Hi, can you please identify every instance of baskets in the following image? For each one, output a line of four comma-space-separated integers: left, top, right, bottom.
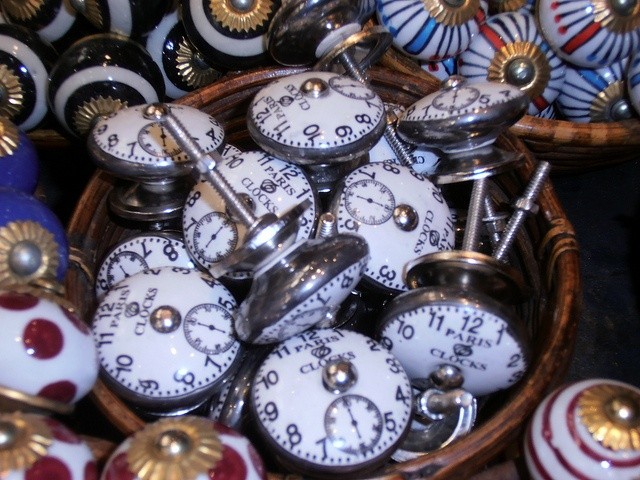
60, 68, 580, 480
363, 1, 639, 176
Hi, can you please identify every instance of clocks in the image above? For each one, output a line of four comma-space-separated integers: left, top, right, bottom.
211, 201, 369, 344
331, 161, 456, 294
395, 75, 530, 183
373, 287, 534, 398
93, 267, 241, 415
182, 152, 321, 281
95, 234, 194, 303
86, 103, 227, 222
252, 326, 416, 480
245, 71, 388, 165
